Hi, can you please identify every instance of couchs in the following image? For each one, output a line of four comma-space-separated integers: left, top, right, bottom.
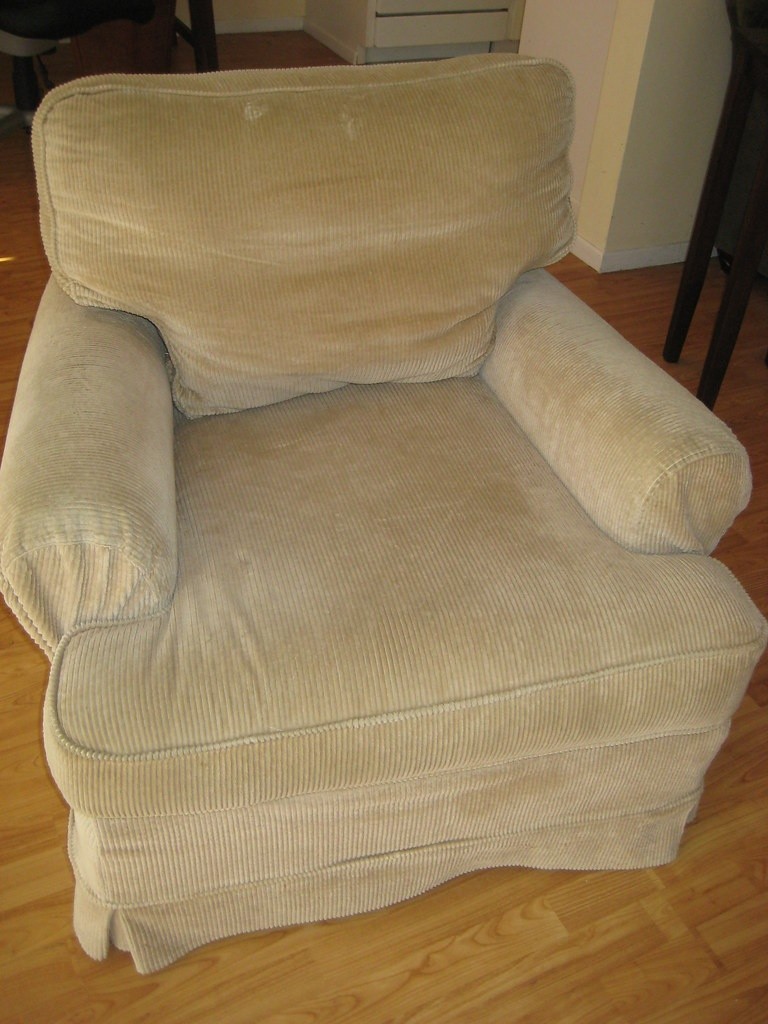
0, 52, 768, 975
